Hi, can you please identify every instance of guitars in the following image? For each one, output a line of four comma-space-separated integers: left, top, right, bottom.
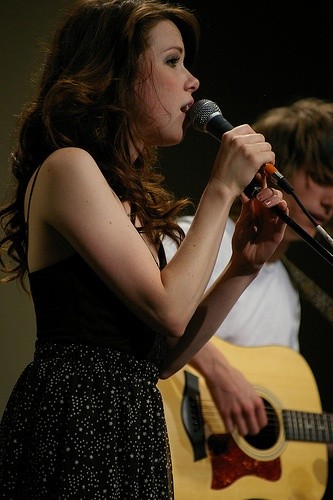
156, 337, 332, 500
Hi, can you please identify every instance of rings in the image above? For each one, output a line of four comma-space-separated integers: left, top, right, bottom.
270, 187, 278, 198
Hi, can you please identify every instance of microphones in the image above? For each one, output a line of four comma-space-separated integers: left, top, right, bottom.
187, 99, 294, 194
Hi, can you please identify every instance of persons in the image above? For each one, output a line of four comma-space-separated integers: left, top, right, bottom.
150, 100, 333, 436
0, 0, 289, 500
